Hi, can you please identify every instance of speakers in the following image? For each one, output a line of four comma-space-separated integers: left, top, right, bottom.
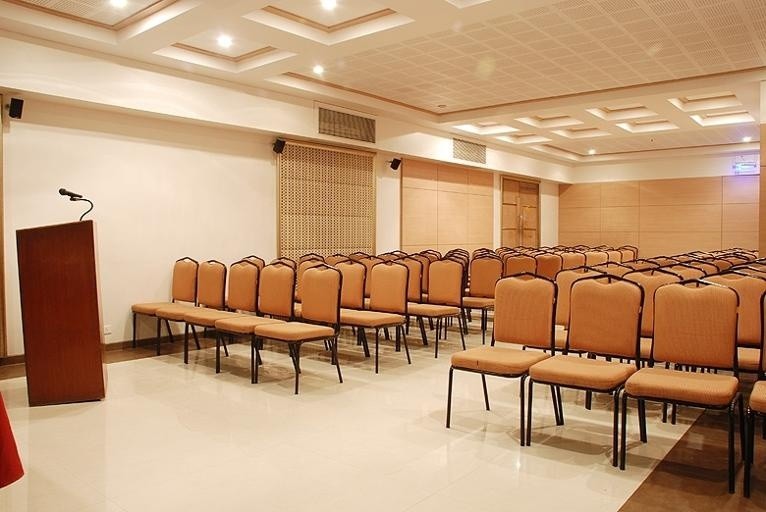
273, 137, 286, 153
8, 98, 24, 120
390, 158, 401, 170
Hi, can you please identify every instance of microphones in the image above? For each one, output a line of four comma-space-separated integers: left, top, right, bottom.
58, 187, 81, 199
